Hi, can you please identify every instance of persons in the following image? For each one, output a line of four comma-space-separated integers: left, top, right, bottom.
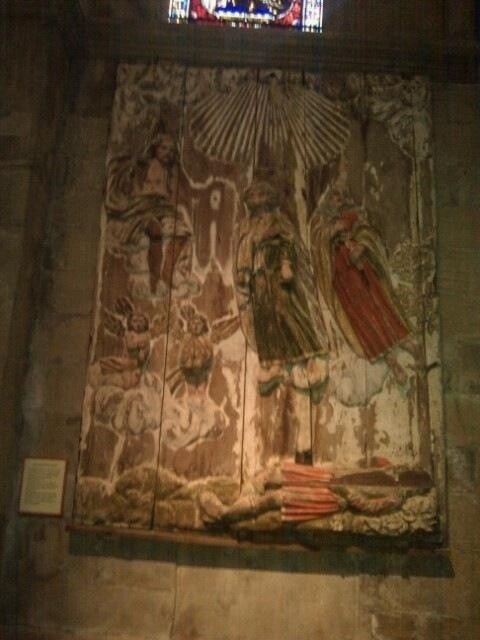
307, 182, 421, 384
166, 304, 241, 450
107, 130, 193, 294
93, 297, 174, 434
233, 182, 332, 406
198, 467, 434, 533
